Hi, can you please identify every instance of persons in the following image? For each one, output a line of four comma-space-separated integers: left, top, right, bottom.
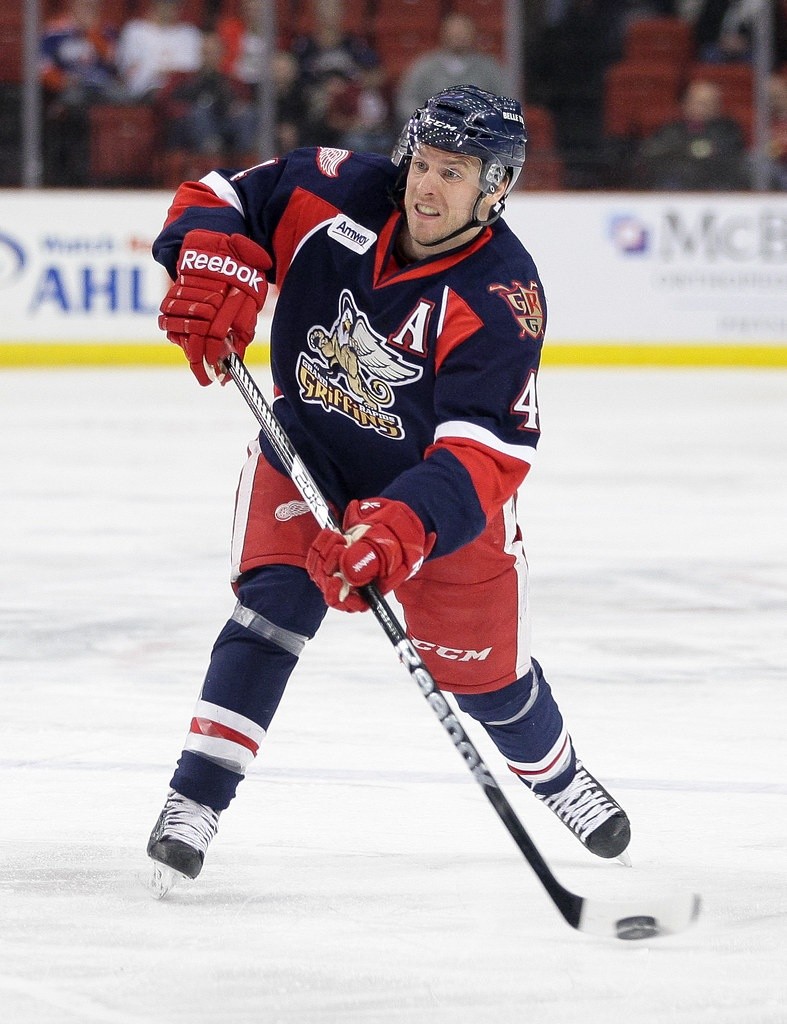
38, 0, 787, 192
148, 86, 630, 900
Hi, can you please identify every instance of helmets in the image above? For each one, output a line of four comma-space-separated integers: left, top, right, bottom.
412, 83, 526, 201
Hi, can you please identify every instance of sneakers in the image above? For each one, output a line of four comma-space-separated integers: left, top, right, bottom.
146, 790, 221, 900
524, 758, 632, 869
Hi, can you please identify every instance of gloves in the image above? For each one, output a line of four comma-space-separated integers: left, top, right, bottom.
158, 228, 273, 387
305, 495, 436, 612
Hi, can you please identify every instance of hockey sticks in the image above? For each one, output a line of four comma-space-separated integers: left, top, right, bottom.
219, 351, 707, 943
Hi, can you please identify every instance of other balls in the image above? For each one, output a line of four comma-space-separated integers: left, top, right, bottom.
616, 915, 657, 940
